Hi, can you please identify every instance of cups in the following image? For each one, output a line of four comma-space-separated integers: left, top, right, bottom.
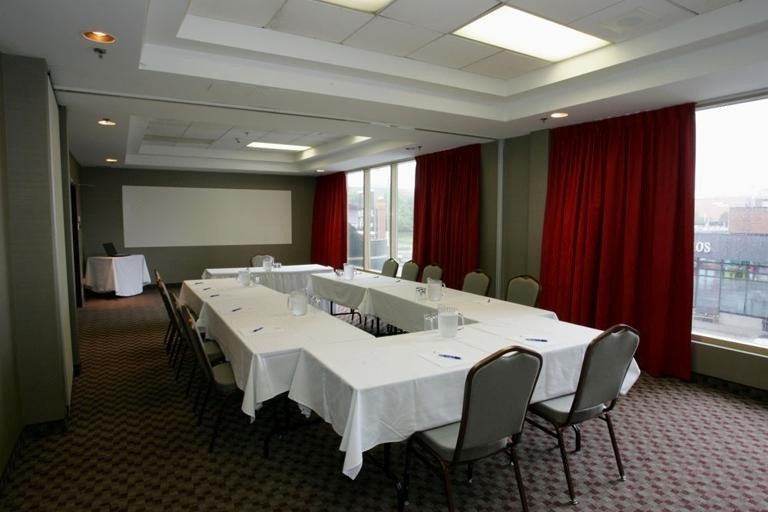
343, 263, 358, 280
263, 257, 273, 271
236, 268, 250, 286
286, 287, 309, 316
423, 313, 438, 333
335, 268, 343, 279
274, 263, 281, 270
436, 304, 464, 338
427, 277, 447, 301
416, 286, 426, 299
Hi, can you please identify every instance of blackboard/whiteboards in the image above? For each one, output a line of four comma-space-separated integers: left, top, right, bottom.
121, 185, 292, 246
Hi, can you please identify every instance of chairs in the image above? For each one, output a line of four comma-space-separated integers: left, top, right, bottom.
181, 305, 251, 455
421, 264, 443, 285
159, 282, 204, 381
504, 275, 539, 306
250, 251, 276, 268
169, 292, 224, 411
508, 324, 640, 505
154, 269, 173, 350
371, 262, 419, 332
463, 271, 490, 295
399, 345, 542, 512
351, 258, 401, 326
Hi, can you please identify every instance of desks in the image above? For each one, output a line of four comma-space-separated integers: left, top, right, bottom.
176, 276, 376, 432
307, 268, 559, 332
201, 261, 333, 312
287, 315, 640, 478
85, 254, 151, 298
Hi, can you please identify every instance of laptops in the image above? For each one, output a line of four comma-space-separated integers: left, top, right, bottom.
97, 240, 129, 256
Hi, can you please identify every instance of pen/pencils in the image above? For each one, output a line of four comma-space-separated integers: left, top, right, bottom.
488, 298, 491, 304
439, 354, 461, 359
196, 282, 203, 284
253, 327, 262, 332
211, 295, 219, 297
527, 339, 547, 342
204, 288, 211, 290
233, 308, 240, 311
